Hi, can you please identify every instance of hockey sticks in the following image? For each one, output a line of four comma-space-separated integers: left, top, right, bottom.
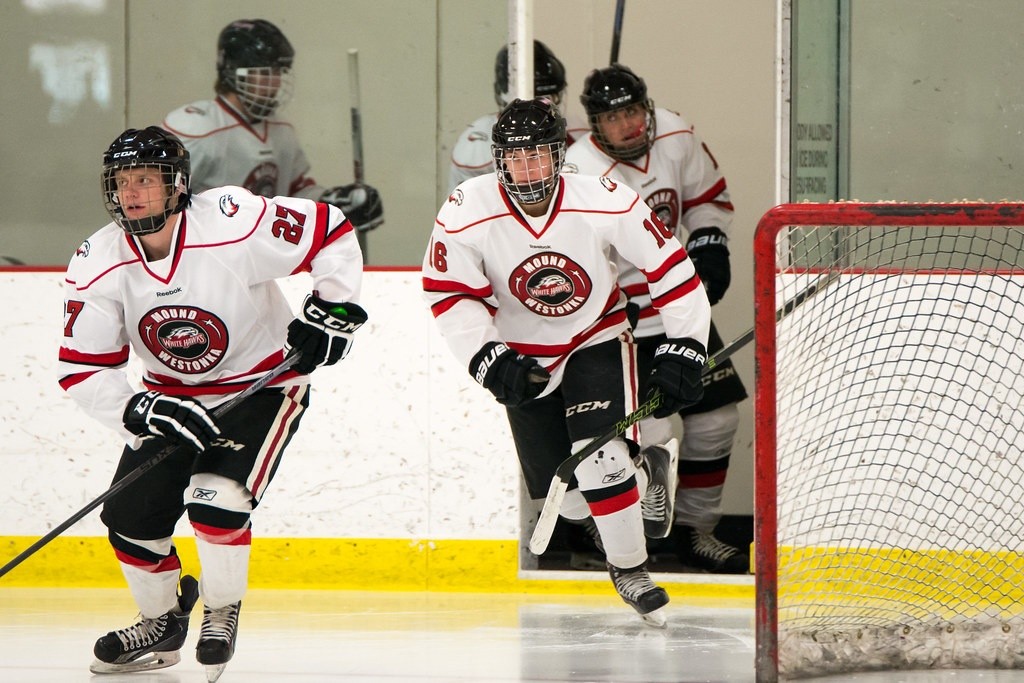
528, 269, 843, 555
346, 48, 368, 265
0, 350, 302, 577
610, 0, 625, 67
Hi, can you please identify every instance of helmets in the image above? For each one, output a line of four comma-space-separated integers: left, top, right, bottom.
490, 97, 568, 206
580, 64, 657, 159
99, 125, 194, 236
215, 19, 295, 123
494, 38, 568, 115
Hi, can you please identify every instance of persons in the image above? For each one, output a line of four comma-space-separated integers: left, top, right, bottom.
422, 97, 713, 629
450, 35, 609, 571
161, 21, 386, 230
56, 126, 368, 683
559, 63, 749, 571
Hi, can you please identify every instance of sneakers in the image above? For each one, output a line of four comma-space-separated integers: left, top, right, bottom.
632, 436, 679, 539
666, 522, 750, 575
194, 598, 241, 683
536, 511, 658, 572
594, 532, 669, 628
89, 574, 200, 673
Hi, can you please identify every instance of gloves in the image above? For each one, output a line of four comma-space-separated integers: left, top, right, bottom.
468, 342, 550, 407
644, 337, 708, 420
686, 226, 733, 306
283, 294, 369, 373
318, 185, 386, 231
122, 391, 221, 454
